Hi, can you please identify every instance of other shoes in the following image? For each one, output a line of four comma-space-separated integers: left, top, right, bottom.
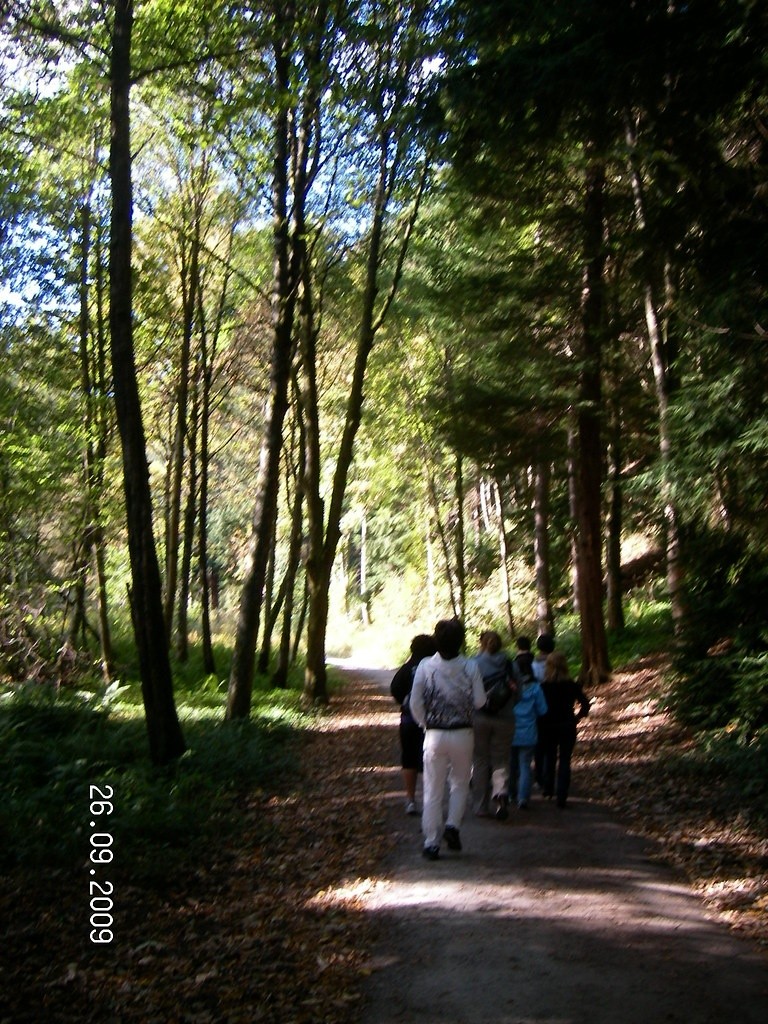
509, 796, 528, 810
541, 788, 567, 809
443, 825, 461, 850
403, 799, 417, 815
493, 802, 508, 822
421, 847, 439, 862
474, 810, 489, 818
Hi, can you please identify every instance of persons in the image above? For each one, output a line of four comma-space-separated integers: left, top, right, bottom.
506, 659, 548, 808
470, 630, 524, 819
531, 635, 554, 683
389, 633, 435, 814
411, 620, 487, 860
511, 637, 535, 659
533, 635, 589, 806
